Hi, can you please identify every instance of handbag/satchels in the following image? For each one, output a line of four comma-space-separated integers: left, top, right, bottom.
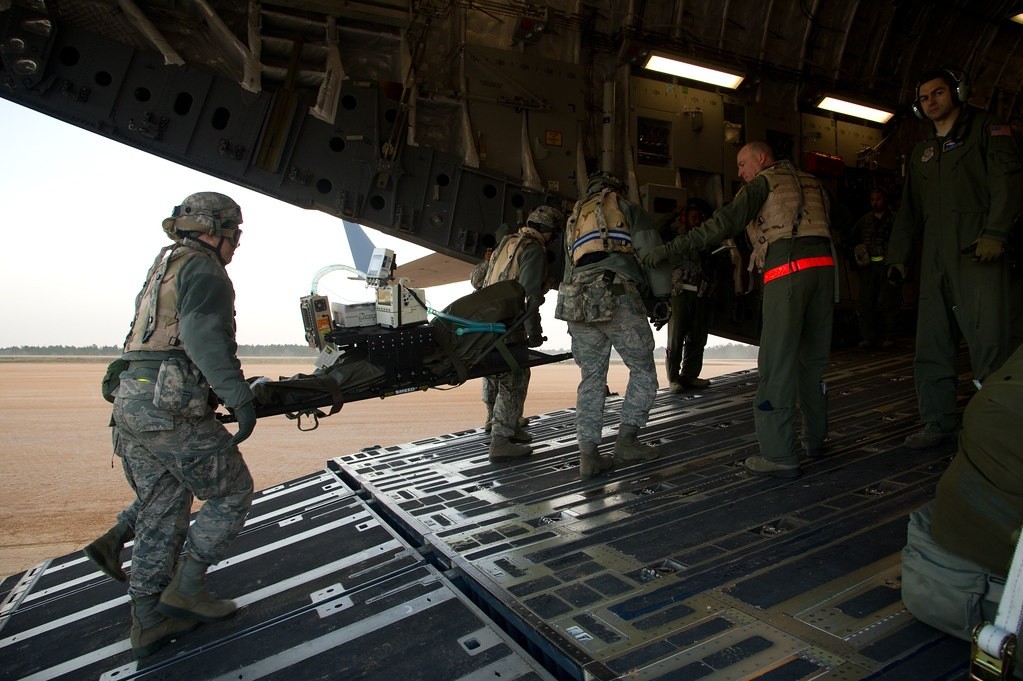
900, 507, 993, 643
555, 282, 616, 323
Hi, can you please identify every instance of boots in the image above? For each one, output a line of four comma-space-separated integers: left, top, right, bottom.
578, 442, 611, 479
156, 555, 238, 622
486, 415, 534, 441
83, 521, 133, 582
612, 425, 663, 465
487, 432, 532, 462
129, 597, 198, 659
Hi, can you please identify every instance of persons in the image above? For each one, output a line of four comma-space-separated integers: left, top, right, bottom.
641, 141, 835, 482
901, 343, 1023, 681
81, 192, 258, 659
471, 205, 566, 463
666, 196, 730, 394
556, 169, 671, 480
855, 51, 1023, 449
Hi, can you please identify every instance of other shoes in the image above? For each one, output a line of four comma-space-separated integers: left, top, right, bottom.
683, 377, 713, 387
668, 380, 682, 393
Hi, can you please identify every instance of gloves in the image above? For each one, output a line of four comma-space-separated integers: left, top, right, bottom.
638, 246, 668, 270
232, 400, 257, 444
526, 328, 544, 348
888, 264, 906, 287
649, 301, 672, 331
968, 236, 1005, 264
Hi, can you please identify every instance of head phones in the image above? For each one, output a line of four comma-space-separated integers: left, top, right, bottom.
911, 68, 966, 119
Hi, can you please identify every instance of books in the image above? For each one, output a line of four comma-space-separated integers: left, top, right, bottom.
711, 245, 736, 255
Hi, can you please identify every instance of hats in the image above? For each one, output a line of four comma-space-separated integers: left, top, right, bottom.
174, 192, 243, 237
583, 170, 627, 193
526, 205, 564, 231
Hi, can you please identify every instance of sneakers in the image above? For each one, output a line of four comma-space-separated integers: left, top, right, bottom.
802, 442, 823, 457
904, 429, 959, 449
745, 455, 801, 479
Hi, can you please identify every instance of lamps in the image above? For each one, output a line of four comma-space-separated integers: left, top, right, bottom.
642, 50, 746, 90
815, 93, 895, 124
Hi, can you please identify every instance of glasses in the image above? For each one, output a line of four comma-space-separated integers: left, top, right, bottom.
162, 217, 243, 247
